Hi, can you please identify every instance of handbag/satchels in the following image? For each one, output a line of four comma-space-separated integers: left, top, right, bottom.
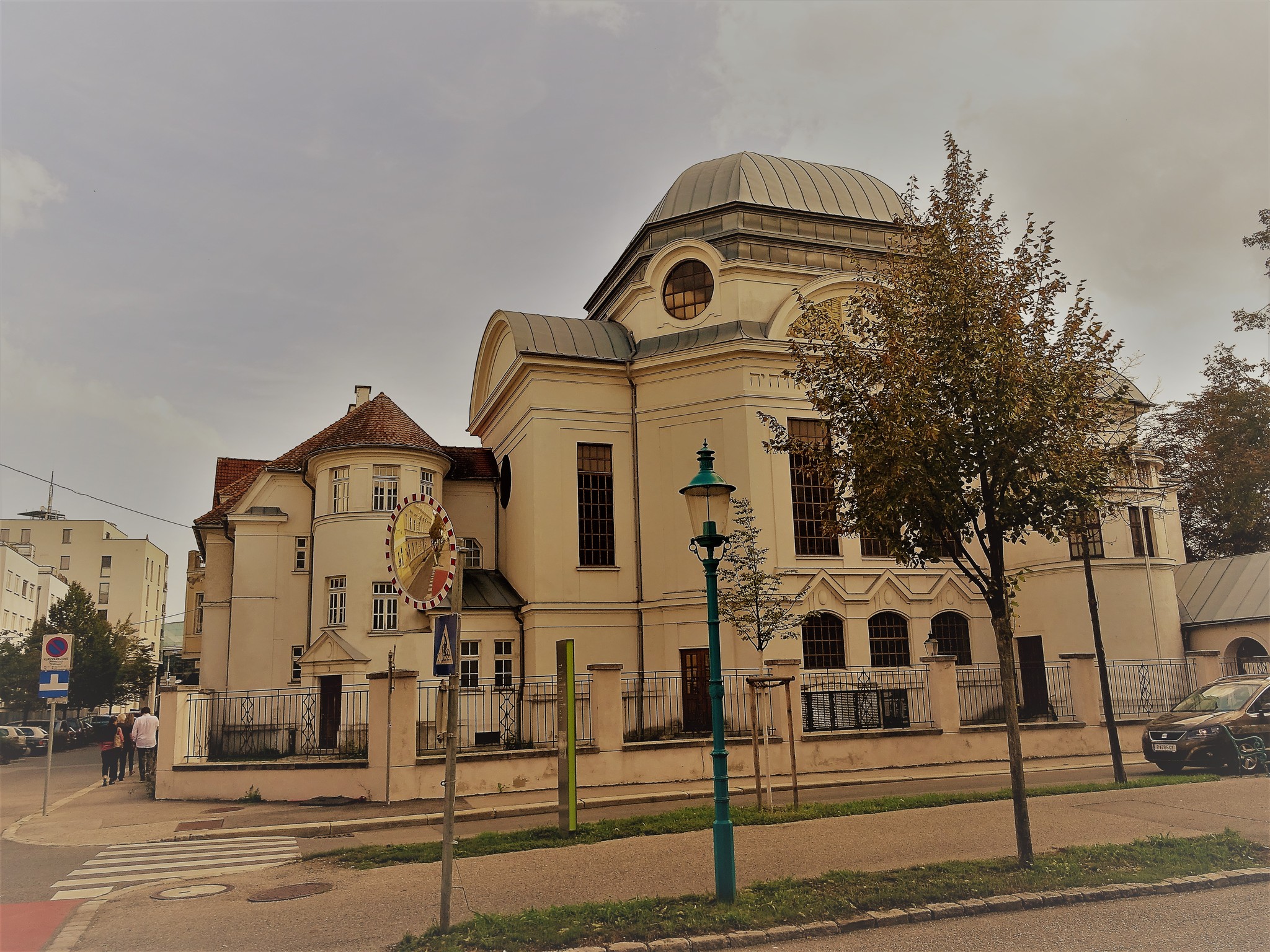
113, 734, 122, 748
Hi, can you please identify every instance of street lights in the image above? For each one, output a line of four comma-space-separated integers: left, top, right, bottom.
678, 437, 736, 906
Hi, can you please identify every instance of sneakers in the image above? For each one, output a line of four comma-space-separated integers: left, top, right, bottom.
129, 771, 136, 776
102, 783, 108, 787
119, 779, 124, 782
108, 781, 115, 785
146, 773, 151, 782
139, 775, 145, 783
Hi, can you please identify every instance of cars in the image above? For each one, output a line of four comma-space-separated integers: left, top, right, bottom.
0, 729, 27, 759
20, 737, 48, 758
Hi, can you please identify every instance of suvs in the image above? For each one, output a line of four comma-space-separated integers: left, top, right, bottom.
65, 711, 141, 749
1141, 673, 1270, 774
16, 726, 50, 739
1, 718, 69, 751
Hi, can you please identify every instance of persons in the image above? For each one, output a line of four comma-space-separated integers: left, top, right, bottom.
114, 706, 161, 781
98, 716, 124, 786
